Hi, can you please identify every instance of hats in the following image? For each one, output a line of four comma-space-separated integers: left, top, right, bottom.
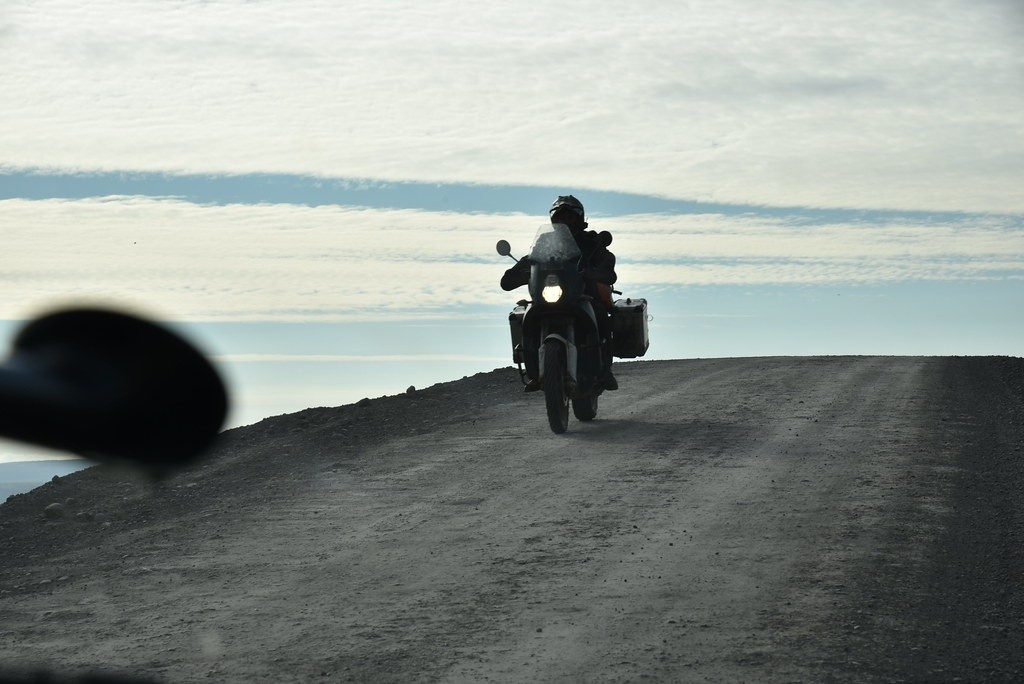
550, 195, 584, 220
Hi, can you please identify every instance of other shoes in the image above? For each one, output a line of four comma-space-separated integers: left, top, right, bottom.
605, 371, 622, 389
524, 379, 542, 392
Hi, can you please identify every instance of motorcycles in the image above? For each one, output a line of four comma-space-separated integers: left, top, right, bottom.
495, 224, 650, 435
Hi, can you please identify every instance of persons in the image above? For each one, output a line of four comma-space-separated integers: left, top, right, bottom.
501, 194, 619, 391
